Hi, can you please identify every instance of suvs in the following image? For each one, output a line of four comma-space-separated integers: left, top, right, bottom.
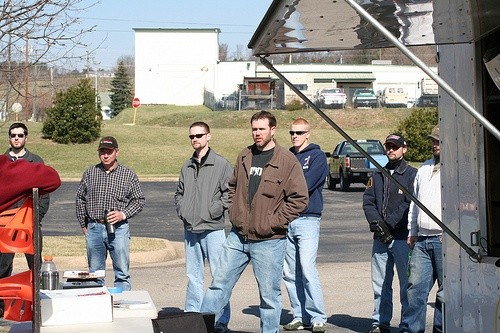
316, 87, 345, 107
351, 88, 378, 108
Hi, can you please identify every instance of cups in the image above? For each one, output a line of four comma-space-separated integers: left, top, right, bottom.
42, 271, 58, 289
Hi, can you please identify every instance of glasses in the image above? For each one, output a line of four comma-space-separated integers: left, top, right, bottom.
189, 133, 208, 140
11, 134, 25, 138
386, 146, 401, 150
289, 130, 309, 136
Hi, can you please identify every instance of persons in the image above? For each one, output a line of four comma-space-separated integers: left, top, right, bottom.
404, 121, 447, 333
362, 132, 422, 333
198, 107, 309, 333
279, 117, 328, 333
75, 136, 145, 291
0, 123, 50, 315
174, 122, 243, 331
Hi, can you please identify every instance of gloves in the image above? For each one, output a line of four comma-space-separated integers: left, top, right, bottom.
381, 231, 394, 244
370, 220, 389, 239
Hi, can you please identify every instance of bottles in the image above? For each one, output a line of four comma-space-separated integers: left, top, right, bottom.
41, 255, 55, 274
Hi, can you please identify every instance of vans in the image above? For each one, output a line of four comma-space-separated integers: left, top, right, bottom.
382, 86, 408, 107
277, 80, 314, 108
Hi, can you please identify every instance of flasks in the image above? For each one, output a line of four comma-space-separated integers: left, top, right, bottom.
105, 207, 115, 235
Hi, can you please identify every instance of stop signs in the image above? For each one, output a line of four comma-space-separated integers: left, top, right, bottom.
132, 97, 140, 108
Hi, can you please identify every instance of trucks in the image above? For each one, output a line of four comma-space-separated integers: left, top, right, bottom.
417, 76, 439, 106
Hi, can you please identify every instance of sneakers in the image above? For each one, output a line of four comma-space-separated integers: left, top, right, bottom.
368, 326, 390, 333
283, 318, 313, 330
312, 322, 327, 332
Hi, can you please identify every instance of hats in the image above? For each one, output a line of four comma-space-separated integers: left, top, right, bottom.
96, 136, 119, 151
426, 124, 439, 141
383, 134, 408, 146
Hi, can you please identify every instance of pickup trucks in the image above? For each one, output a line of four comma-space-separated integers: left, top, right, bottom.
323, 138, 388, 191
